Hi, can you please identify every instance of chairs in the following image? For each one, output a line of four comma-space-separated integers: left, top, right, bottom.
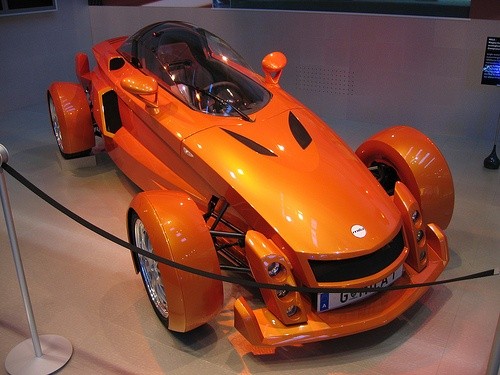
151, 35, 216, 109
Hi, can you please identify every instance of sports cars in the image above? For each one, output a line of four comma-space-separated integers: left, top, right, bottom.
44, 17, 456, 359
